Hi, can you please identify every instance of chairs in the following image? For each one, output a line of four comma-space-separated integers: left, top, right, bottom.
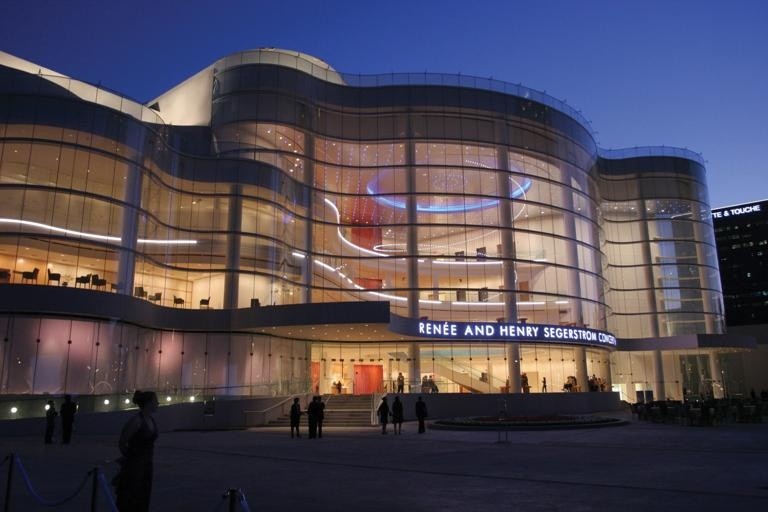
21, 268, 118, 294
635, 399, 766, 426
134, 287, 212, 309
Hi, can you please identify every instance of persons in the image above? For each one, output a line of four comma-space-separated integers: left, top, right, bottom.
290, 397, 304, 439
541, 376, 547, 392
307, 395, 316, 438
561, 375, 609, 394
377, 396, 391, 434
44, 401, 58, 443
414, 396, 429, 433
397, 371, 404, 393
315, 396, 325, 438
392, 396, 404, 435
118, 390, 159, 512
336, 380, 341, 393
422, 373, 439, 393
60, 394, 76, 445
